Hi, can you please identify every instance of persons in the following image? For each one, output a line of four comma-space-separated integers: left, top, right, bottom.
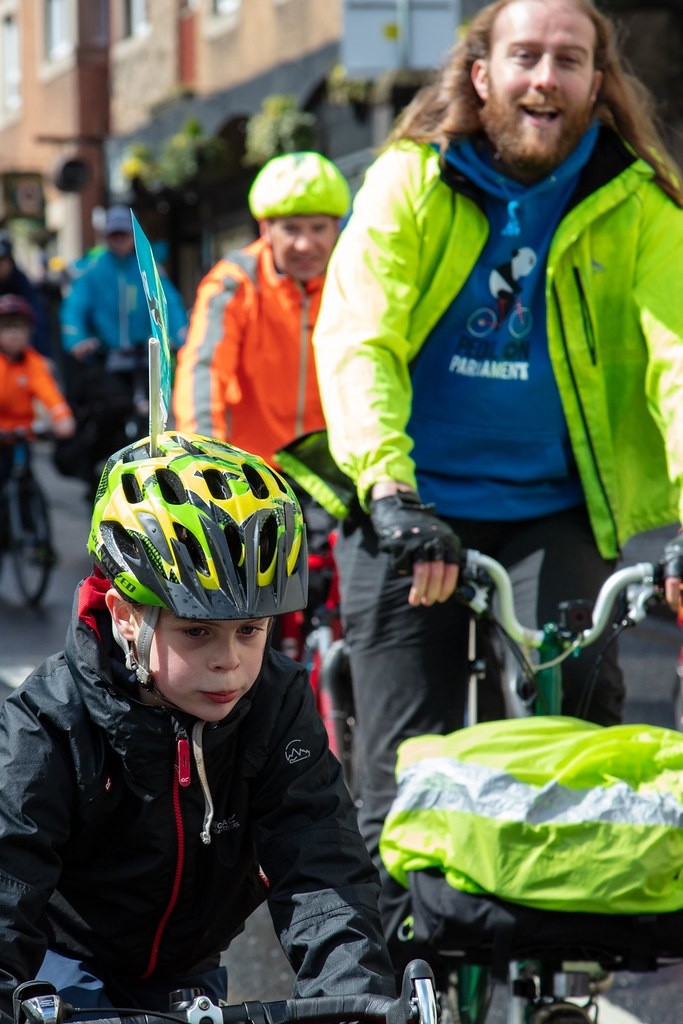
0, 433, 397, 1024
0, 296, 74, 569
170, 150, 354, 628
0, 238, 44, 350
60, 206, 188, 502
314, 0, 683, 1024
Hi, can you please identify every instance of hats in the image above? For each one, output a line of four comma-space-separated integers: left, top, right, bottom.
101, 205, 135, 236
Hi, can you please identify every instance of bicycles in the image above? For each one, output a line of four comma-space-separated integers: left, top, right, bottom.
283, 520, 358, 809
1, 960, 441, 1024
1, 423, 56, 610
74, 340, 180, 517
387, 542, 683, 1023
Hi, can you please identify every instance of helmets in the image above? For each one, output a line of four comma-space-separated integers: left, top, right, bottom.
0, 295, 35, 324
85, 431, 309, 620
250, 151, 352, 219
